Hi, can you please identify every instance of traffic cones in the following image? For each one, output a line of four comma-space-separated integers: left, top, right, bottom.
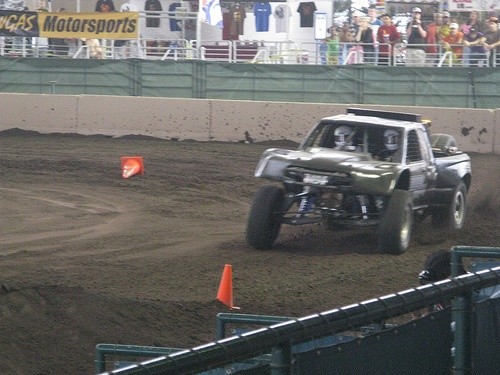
215, 263, 239, 311
120, 156, 145, 180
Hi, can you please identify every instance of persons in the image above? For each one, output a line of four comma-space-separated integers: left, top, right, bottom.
376, 130, 411, 163
421, 248, 467, 315
320, 5, 500, 68
0, 0, 146, 60
334, 126, 354, 152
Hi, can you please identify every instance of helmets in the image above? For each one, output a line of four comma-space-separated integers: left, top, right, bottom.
333, 126, 354, 146
383, 129, 400, 151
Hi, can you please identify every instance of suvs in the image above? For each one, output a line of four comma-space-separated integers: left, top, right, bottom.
245, 106, 472, 255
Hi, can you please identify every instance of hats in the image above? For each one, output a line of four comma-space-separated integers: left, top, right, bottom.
443, 11, 451, 19
352, 10, 365, 16
449, 22, 459, 30
470, 27, 479, 32
486, 17, 498, 23
411, 8, 422, 13
